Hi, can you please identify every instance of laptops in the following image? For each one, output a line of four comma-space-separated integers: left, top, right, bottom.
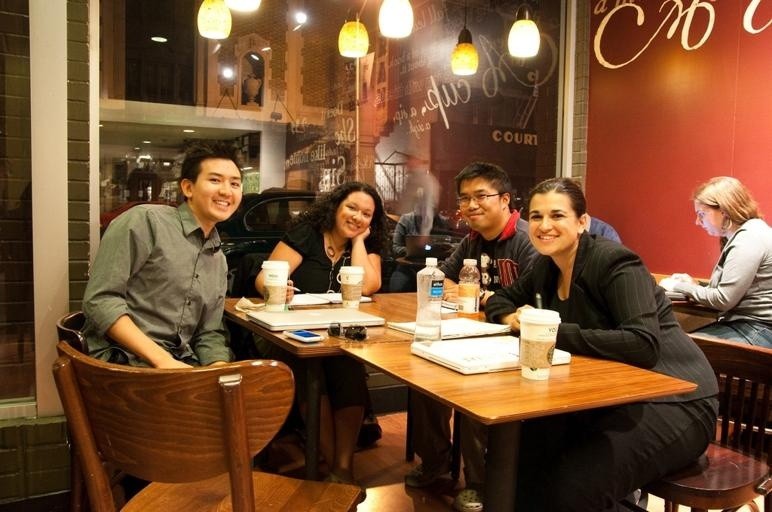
405, 235, 450, 261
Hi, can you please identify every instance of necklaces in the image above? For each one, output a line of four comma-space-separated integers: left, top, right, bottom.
327, 231, 344, 257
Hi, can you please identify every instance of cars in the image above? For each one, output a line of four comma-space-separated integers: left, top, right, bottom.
100, 198, 181, 234
217, 188, 465, 301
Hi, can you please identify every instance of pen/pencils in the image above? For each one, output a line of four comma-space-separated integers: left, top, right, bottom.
535, 292, 542, 308
287, 286, 301, 292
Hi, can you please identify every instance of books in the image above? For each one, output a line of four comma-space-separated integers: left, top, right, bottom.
387, 316, 511, 339
287, 290, 373, 307
656, 275, 687, 301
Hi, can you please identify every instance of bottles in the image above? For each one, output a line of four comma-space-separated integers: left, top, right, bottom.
457, 257, 482, 320
411, 255, 446, 346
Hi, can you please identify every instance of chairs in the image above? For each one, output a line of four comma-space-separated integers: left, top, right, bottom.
637, 334, 772, 512
55, 310, 91, 512
50, 338, 366, 512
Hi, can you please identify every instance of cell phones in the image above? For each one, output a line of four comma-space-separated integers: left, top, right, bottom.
283, 329, 324, 343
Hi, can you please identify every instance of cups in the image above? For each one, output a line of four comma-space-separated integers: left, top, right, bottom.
517, 306, 562, 382
261, 260, 289, 310
339, 265, 365, 311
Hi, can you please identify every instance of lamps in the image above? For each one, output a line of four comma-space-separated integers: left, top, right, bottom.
450, 1, 479, 77
337, 0, 371, 61
196, 0, 234, 40
506, 0, 542, 60
377, 0, 416, 41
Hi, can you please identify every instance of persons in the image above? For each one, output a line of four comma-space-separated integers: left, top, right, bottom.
78, 141, 244, 501
660, 175, 771, 371
98, 163, 179, 236
389, 196, 450, 293
401, 160, 540, 511
252, 180, 392, 485
484, 177, 720, 511
582, 212, 623, 246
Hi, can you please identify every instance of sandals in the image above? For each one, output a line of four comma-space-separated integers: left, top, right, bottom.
324, 472, 366, 503
405, 462, 452, 488
453, 488, 484, 512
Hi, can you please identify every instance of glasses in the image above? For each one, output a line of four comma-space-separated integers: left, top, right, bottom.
328, 324, 369, 341
456, 194, 500, 207
